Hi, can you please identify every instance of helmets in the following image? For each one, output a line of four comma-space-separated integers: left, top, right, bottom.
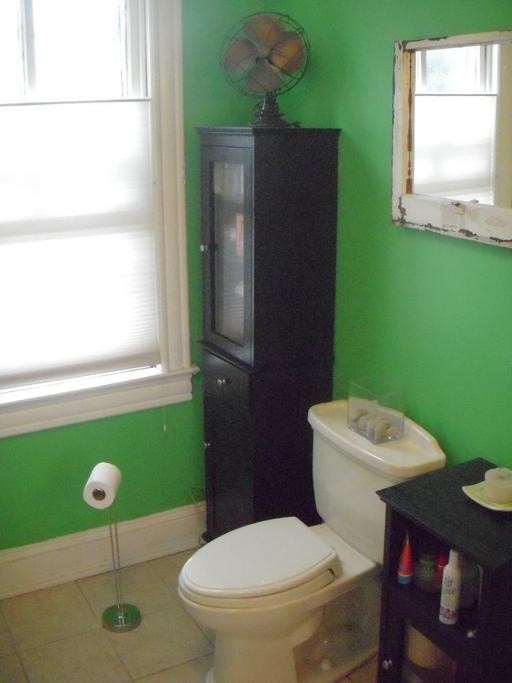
461, 480, 512, 513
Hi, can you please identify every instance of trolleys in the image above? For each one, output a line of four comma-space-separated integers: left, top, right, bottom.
218, 10, 312, 128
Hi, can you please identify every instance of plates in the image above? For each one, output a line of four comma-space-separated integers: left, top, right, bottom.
375, 458, 512, 683
195, 340, 340, 540
193, 126, 344, 366
